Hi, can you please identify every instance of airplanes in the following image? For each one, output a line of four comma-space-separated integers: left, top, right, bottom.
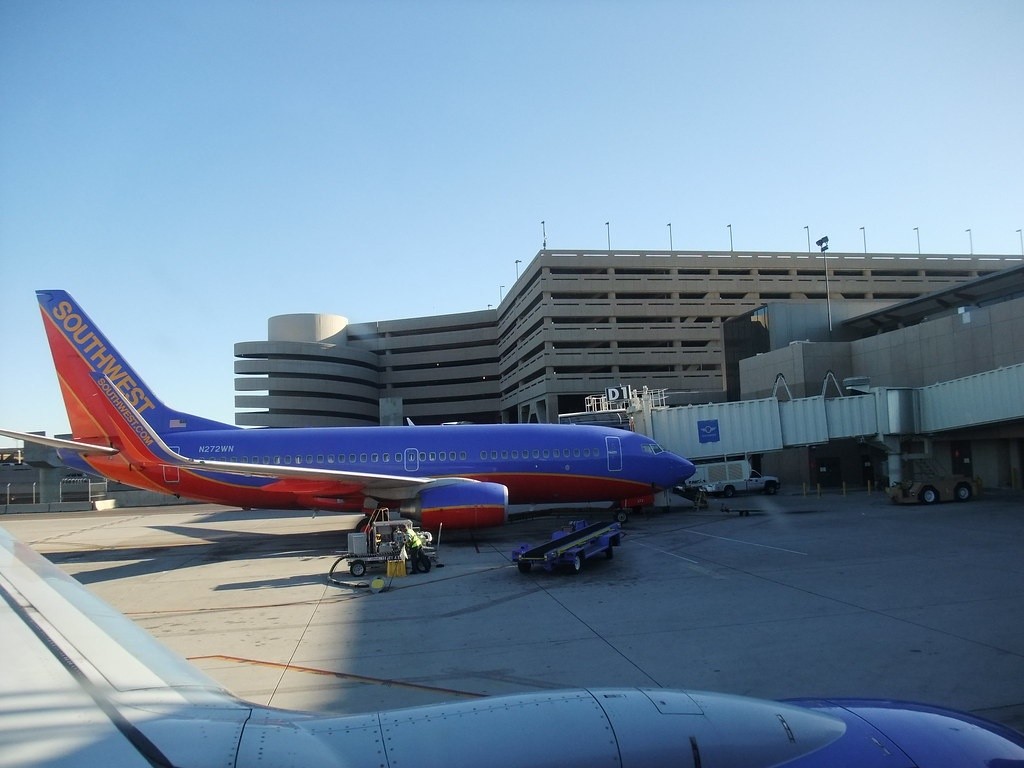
0, 290, 708, 534
0, 530, 1024, 767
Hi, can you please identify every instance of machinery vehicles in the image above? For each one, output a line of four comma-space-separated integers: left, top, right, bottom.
885, 452, 985, 505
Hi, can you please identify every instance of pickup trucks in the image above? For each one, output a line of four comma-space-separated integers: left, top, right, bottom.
698, 469, 781, 498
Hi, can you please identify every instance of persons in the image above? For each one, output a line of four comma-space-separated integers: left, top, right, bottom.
399, 524, 431, 573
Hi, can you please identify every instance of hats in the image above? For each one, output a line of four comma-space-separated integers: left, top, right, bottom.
400, 524, 407, 528
